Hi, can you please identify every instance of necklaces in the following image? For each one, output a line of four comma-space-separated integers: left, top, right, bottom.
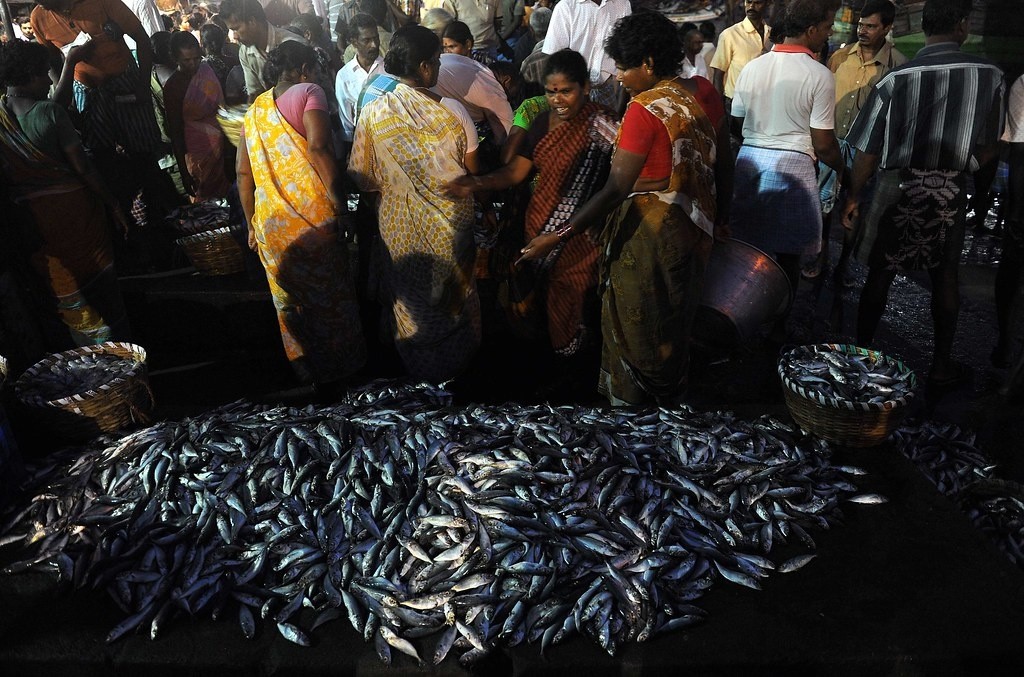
57, 8, 75, 28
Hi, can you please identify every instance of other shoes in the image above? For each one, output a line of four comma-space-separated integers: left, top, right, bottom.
801, 264, 825, 283
832, 275, 857, 290
992, 345, 1014, 369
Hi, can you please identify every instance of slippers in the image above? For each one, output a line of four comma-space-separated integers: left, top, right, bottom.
930, 362, 962, 385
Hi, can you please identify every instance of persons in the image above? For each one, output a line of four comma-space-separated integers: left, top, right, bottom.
514, 9, 732, 406
0, 0, 1024, 504
346, 24, 498, 379
235, 39, 355, 383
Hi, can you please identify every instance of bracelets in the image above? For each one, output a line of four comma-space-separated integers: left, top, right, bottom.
472, 176, 483, 186
333, 214, 349, 216
555, 220, 575, 241
716, 216, 729, 224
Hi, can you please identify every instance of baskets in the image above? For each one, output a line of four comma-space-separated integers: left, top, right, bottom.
778, 342, 914, 445
14, 342, 154, 454
176, 221, 254, 282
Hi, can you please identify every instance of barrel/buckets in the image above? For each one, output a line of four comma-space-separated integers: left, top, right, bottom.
692, 236, 795, 358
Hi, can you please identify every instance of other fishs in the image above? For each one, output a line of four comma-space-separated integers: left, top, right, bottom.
0, 347, 1023, 672
